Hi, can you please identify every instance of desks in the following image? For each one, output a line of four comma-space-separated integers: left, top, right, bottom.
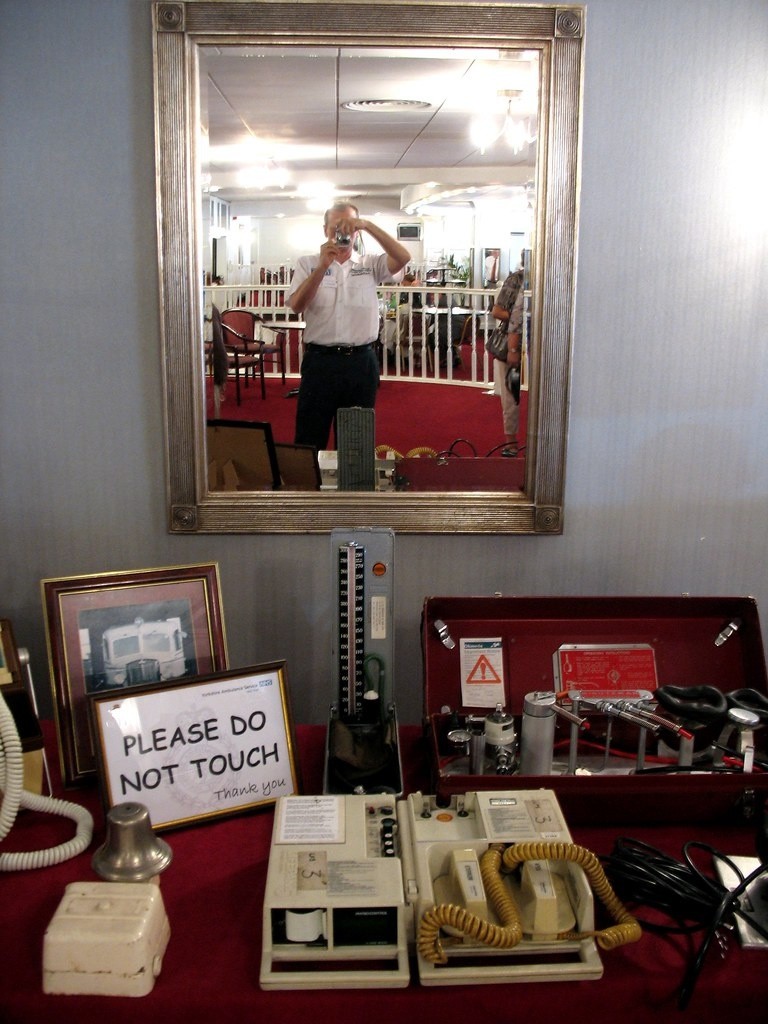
0, 722, 768, 1024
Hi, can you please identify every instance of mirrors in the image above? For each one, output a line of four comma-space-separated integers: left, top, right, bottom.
149, 0, 589, 541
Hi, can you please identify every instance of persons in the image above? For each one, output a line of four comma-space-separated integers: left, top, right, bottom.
210, 264, 291, 308
284, 202, 412, 452
379, 247, 524, 457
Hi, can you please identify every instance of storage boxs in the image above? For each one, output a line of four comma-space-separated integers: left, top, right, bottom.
415, 593, 768, 829
38, 880, 171, 997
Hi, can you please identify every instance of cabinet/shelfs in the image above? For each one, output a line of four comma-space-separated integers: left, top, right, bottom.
421, 247, 467, 295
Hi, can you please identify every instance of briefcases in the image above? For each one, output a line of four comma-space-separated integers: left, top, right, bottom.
419, 594, 768, 826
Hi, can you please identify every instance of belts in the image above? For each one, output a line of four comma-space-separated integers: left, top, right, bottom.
488, 280, 497, 283
306, 343, 371, 356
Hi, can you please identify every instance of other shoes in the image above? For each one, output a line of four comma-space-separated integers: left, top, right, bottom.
501, 448, 518, 456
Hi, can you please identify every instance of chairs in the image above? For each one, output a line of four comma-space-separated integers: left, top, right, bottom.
452, 316, 484, 371
203, 316, 267, 406
221, 308, 287, 387
399, 312, 433, 372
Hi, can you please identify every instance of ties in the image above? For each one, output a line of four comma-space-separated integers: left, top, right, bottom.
490, 257, 497, 283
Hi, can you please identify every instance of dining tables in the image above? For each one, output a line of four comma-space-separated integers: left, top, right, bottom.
380, 314, 432, 370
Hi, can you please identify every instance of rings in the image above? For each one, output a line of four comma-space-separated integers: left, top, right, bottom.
323, 244, 328, 248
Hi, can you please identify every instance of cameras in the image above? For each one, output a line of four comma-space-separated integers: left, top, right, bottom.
336, 230, 352, 247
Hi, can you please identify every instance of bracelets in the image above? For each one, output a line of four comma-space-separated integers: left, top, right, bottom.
509, 348, 519, 354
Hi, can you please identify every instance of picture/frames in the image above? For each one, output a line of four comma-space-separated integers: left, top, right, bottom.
40, 560, 229, 795
203, 416, 282, 490
84, 657, 300, 836
274, 441, 322, 492
0, 618, 25, 693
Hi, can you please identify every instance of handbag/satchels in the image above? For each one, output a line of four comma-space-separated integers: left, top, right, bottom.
485, 330, 508, 363
